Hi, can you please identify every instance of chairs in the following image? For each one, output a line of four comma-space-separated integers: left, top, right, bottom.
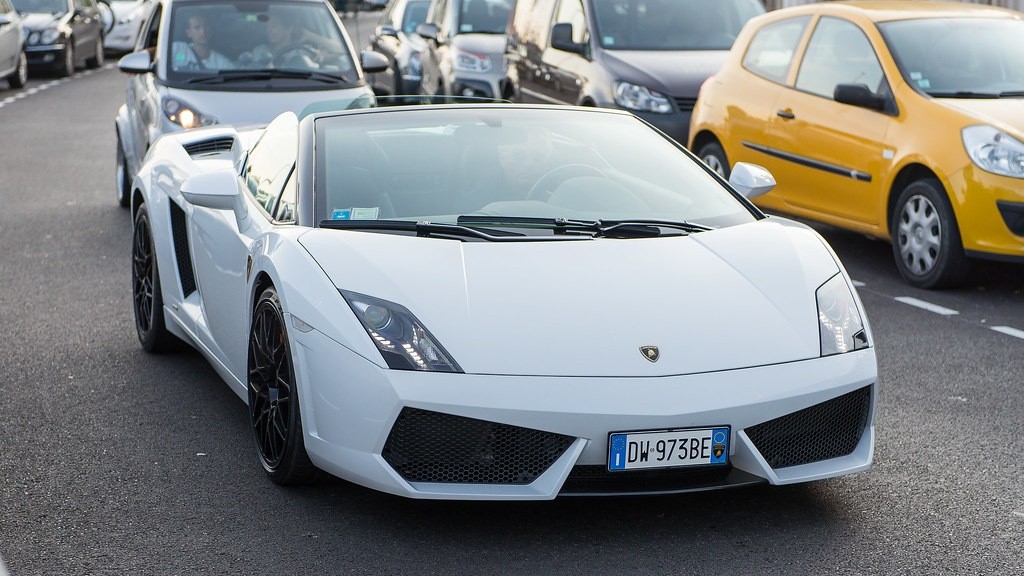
326, 129, 396, 219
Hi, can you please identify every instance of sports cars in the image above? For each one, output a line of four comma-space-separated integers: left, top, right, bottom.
130, 94, 878, 500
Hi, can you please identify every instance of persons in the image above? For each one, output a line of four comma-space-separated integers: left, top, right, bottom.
238, 11, 345, 68
137, 14, 231, 69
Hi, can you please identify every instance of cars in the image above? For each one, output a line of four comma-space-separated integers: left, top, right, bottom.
114, 0, 391, 208
499, 1, 786, 146
324, 0, 513, 108
687, 1, 1024, 290
0, 0, 159, 88
